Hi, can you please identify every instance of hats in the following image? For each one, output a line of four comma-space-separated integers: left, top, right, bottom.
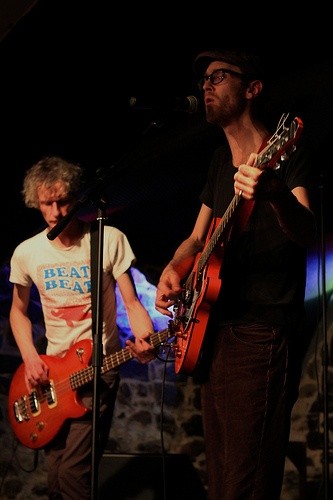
193, 49, 261, 83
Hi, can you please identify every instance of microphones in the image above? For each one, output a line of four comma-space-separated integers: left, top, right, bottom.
129, 95, 199, 115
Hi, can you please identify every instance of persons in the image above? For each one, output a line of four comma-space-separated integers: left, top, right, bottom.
9, 156, 156, 499
154, 51, 319, 499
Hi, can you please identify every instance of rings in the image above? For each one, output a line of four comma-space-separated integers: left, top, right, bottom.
239, 190, 242, 196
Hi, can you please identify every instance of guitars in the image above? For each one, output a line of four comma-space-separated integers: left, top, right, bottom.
172, 113, 305, 376
7, 325, 177, 450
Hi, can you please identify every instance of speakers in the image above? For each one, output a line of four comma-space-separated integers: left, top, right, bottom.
97, 453, 208, 500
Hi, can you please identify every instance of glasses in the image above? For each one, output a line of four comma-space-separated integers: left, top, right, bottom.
201, 69, 255, 85
